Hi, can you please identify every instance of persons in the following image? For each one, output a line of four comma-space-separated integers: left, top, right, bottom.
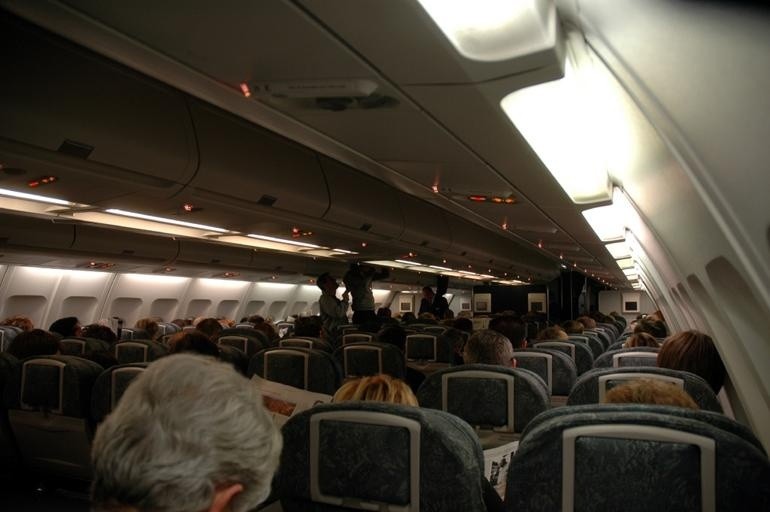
67, 353, 275, 512
418, 286, 450, 315
330, 372, 503, 508
316, 271, 350, 327
344, 260, 390, 320
1, 305, 729, 411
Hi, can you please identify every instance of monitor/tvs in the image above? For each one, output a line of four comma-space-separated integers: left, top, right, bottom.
152, 323, 196, 347
19, 358, 66, 415
598, 372, 684, 405
404, 323, 470, 356
308, 410, 419, 511
111, 368, 146, 412
279, 338, 312, 350
220, 321, 295, 339
561, 423, 715, 511
115, 342, 147, 364
512, 352, 552, 396
115, 328, 133, 340
613, 351, 662, 368
263, 349, 308, 390
218, 336, 248, 354
343, 345, 382, 378
60, 338, 85, 355
441, 369, 514, 433
406, 334, 437, 362
532, 322, 664, 362
472, 317, 492, 329
337, 323, 372, 345
